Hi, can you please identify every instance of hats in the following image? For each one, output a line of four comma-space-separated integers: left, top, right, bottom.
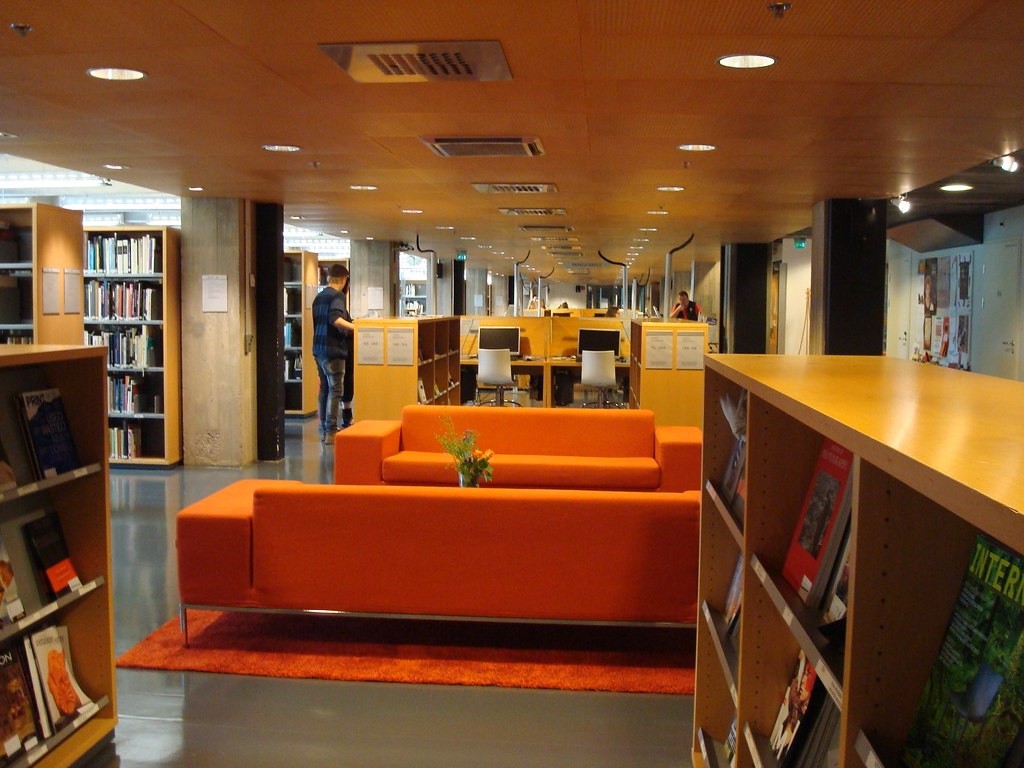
328, 264, 349, 277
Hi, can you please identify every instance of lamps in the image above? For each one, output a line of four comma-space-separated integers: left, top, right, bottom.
97, 175, 112, 187
990, 156, 1016, 172
891, 195, 910, 213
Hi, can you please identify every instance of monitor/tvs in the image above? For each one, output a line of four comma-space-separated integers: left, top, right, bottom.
577, 328, 621, 359
478, 326, 520, 355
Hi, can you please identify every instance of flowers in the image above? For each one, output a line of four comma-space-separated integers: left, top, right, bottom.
434, 413, 495, 488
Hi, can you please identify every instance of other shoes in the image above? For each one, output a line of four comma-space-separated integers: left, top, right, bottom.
325, 432, 339, 444
320, 429, 326, 441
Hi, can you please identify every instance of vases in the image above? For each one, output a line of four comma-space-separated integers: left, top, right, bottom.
457, 471, 479, 488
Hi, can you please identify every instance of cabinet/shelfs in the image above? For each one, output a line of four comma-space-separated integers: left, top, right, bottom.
692, 353, 1024, 768
282, 250, 461, 421
0, 201, 177, 768
628, 318, 708, 428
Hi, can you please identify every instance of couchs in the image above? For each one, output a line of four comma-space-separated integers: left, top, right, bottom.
334, 404, 703, 495
176, 478, 700, 648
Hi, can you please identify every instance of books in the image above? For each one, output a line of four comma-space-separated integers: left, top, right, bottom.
285, 319, 299, 347
111, 280, 160, 321
723, 714, 737, 768
285, 360, 293, 379
87, 232, 117, 273
0, 437, 18, 493
107, 375, 161, 414
117, 234, 162, 273
781, 436, 854, 623
84, 325, 124, 368
109, 420, 142, 459
84, 280, 111, 320
406, 300, 423, 315
769, 649, 841, 768
12, 388, 81, 482
0, 512, 101, 768
405, 284, 416, 296
121, 324, 163, 369
284, 287, 298, 315
894, 535, 1024, 768
7, 337, 32, 344
720, 440, 746, 524
721, 552, 744, 637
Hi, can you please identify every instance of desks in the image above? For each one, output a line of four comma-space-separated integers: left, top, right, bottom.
460, 308, 638, 409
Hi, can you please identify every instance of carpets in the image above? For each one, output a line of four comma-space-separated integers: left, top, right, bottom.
115, 608, 694, 695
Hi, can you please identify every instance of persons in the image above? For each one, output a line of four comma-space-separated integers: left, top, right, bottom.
669, 291, 702, 321
312, 264, 354, 446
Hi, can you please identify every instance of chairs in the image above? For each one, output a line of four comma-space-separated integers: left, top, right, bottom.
476, 348, 523, 408
580, 350, 627, 410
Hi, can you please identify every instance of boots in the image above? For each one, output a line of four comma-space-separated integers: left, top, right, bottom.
341, 408, 355, 427
